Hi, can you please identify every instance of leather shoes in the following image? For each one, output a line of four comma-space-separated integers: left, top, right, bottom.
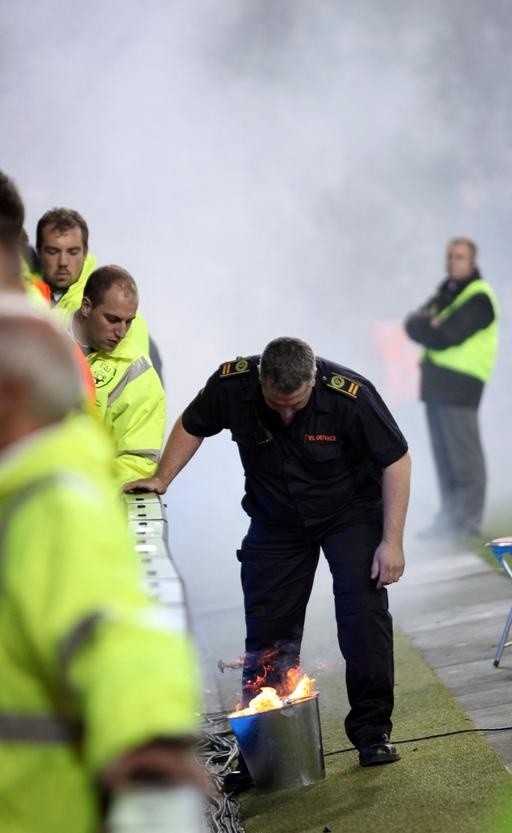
222, 761, 252, 792
355, 732, 401, 766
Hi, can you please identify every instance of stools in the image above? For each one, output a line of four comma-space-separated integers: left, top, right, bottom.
483, 536, 512, 668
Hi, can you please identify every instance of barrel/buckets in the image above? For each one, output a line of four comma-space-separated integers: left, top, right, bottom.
228, 691, 327, 794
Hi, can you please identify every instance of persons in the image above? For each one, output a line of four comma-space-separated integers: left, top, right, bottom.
34, 203, 150, 359
61, 262, 168, 516
119, 335, 415, 768
0, 169, 65, 331
0, 313, 234, 833
404, 235, 502, 542
